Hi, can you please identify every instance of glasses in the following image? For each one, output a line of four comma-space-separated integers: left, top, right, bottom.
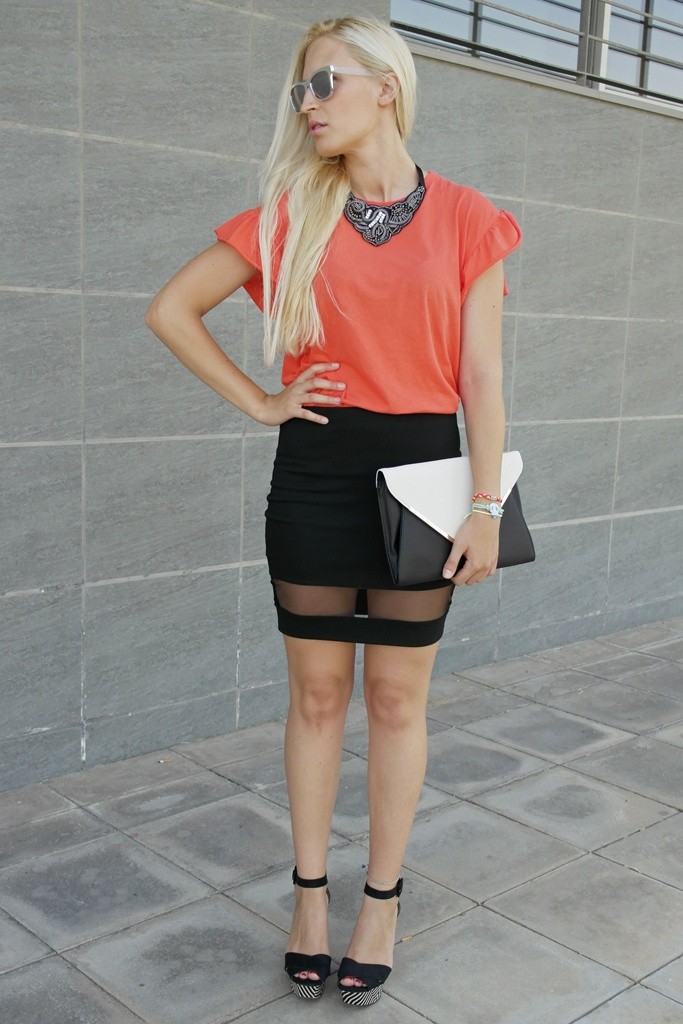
289, 65, 383, 115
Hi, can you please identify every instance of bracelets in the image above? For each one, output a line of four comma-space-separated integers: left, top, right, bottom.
468, 493, 506, 520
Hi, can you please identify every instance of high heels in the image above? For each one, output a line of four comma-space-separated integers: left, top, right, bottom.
284, 867, 331, 999
338, 878, 405, 1005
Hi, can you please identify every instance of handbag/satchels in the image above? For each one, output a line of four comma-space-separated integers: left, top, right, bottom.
375, 450, 537, 590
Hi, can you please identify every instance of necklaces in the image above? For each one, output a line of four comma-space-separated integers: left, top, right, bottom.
339, 164, 427, 247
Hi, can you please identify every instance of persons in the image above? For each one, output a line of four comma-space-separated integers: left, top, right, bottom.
143, 11, 521, 1008
